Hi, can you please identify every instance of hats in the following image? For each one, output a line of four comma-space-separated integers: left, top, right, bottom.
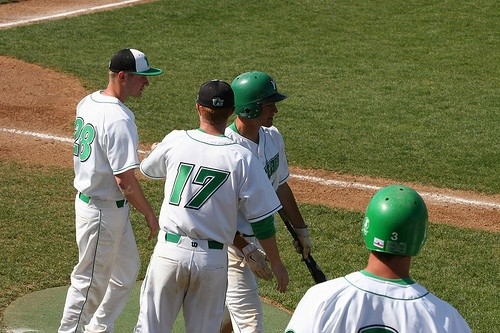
197, 79, 235, 109
109, 48, 162, 76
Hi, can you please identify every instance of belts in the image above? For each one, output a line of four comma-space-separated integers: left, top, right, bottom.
237, 231, 255, 237
165, 232, 224, 250
79, 193, 128, 208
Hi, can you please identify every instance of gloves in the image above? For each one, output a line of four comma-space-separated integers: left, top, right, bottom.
292, 226, 311, 262
240, 242, 274, 282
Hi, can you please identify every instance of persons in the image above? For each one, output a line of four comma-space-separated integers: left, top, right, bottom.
58, 48, 162, 333
133, 81, 289, 333
284, 184, 471, 333
221, 72, 311, 333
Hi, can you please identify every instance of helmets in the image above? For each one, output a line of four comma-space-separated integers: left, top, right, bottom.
361, 185, 429, 256
230, 72, 287, 118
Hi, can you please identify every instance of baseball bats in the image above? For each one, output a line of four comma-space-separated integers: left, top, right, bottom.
276, 209, 327, 283
